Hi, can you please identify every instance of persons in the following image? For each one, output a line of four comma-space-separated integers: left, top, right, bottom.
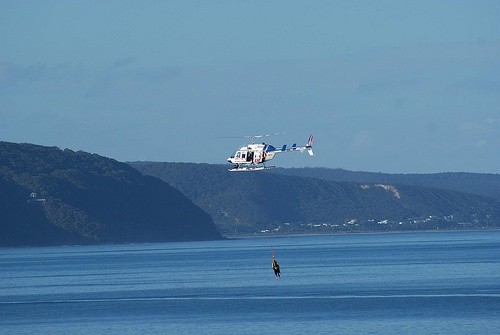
272, 260, 281, 280
248, 154, 252, 161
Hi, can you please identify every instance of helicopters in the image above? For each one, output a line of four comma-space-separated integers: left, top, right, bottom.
222, 133, 315, 174
